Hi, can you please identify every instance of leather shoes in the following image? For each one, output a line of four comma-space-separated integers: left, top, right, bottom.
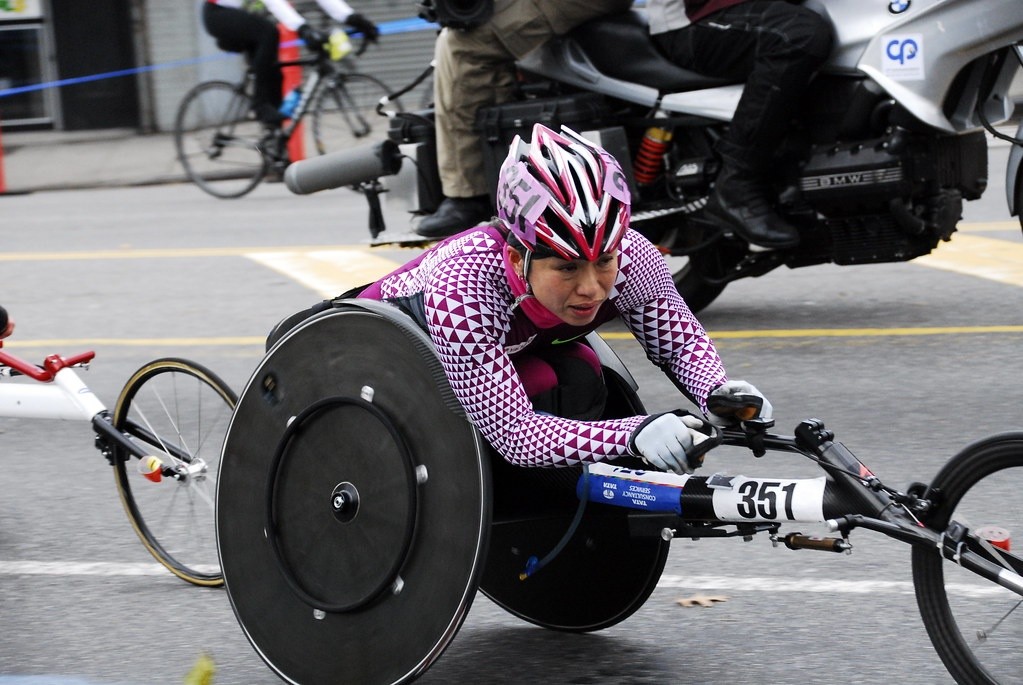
704, 167, 801, 250
416, 194, 490, 238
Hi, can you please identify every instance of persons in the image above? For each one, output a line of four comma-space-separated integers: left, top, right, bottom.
414, 0, 636, 232
645, 0, 838, 247
264, 126, 774, 474
202, 0, 379, 167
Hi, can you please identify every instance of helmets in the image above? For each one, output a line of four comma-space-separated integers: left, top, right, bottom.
495, 122, 630, 263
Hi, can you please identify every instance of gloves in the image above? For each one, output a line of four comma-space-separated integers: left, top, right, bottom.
704, 378, 774, 428
299, 25, 329, 57
636, 410, 703, 476
345, 13, 380, 45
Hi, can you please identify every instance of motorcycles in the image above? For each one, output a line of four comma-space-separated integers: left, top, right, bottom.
283, 0, 1022, 327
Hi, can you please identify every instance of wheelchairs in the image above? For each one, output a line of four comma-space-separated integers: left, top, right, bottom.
0, 307, 240, 589
213, 298, 1023, 685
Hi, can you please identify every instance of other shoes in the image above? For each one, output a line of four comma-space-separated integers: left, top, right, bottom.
258, 135, 293, 180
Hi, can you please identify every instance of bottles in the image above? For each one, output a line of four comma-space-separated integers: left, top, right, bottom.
278, 86, 302, 117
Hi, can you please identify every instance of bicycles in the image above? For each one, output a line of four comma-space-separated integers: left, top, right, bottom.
171, 26, 407, 199
